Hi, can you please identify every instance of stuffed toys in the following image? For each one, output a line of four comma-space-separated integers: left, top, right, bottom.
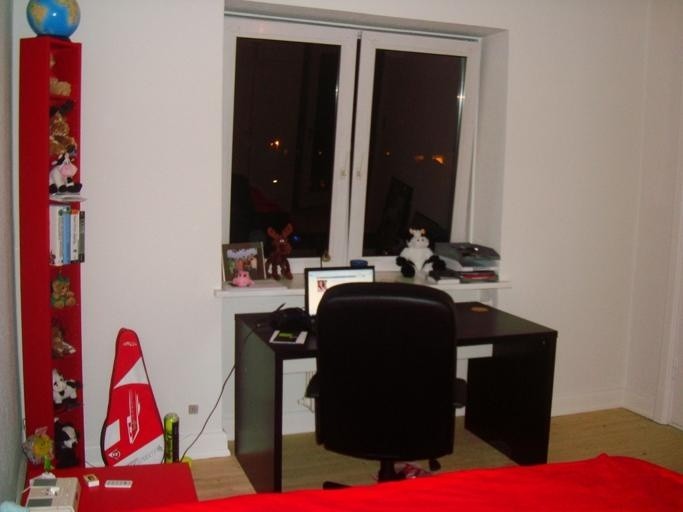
50, 323, 75, 358
49, 271, 75, 309
19, 424, 56, 471
394, 227, 445, 285
49, 75, 71, 96
52, 415, 81, 469
264, 223, 294, 281
49, 100, 79, 161
50, 367, 82, 409
49, 151, 83, 194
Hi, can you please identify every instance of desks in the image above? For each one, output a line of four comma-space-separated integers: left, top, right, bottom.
234, 300, 558, 493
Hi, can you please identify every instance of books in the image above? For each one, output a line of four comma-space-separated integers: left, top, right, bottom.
48, 201, 85, 267
427, 242, 501, 285
49, 192, 88, 203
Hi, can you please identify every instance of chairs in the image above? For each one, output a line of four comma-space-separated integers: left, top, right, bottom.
304, 281, 465, 493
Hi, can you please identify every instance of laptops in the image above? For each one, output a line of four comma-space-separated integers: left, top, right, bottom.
305, 267, 375, 335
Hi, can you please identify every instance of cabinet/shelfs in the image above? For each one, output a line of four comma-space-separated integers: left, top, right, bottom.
21, 37, 85, 473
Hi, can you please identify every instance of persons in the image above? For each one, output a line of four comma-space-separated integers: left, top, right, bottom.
316, 280, 326, 292
226, 252, 261, 278
